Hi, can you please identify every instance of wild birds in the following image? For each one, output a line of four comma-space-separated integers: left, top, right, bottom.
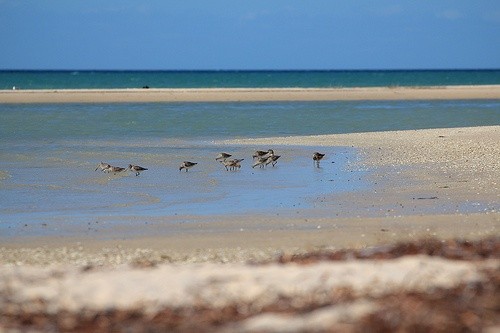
215, 152, 245, 171
252, 148, 281, 168
124, 163, 149, 177
178, 161, 198, 172
94, 161, 127, 175
312, 151, 325, 168
